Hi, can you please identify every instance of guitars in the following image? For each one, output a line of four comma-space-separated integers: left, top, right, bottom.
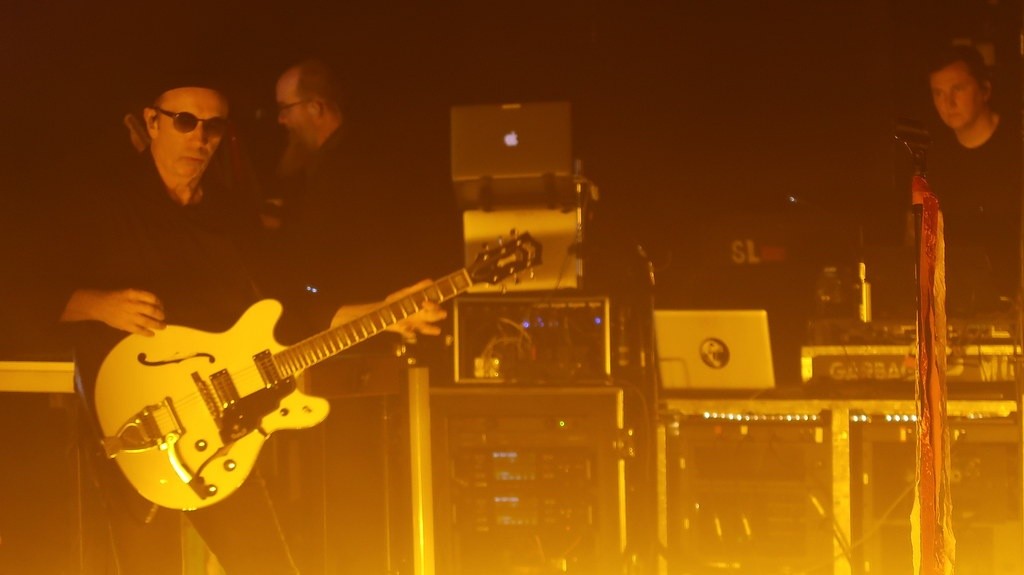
94, 229, 544, 512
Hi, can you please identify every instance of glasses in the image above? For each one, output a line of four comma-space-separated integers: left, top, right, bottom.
147, 101, 230, 138
273, 99, 304, 116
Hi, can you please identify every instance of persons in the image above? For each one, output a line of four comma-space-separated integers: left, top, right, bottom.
27, 60, 454, 572
261, 60, 437, 310
906, 44, 1024, 305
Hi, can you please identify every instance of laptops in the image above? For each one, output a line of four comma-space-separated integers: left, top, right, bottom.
653, 309, 807, 397
450, 100, 575, 213
